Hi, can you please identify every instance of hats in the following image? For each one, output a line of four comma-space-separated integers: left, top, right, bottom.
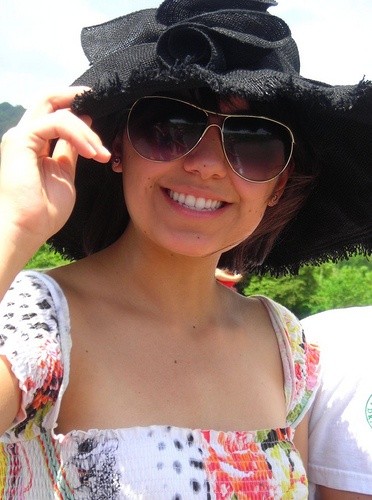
42, 0, 372, 271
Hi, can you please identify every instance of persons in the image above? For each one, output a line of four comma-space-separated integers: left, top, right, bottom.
300, 305, 372, 500
0, 0, 372, 500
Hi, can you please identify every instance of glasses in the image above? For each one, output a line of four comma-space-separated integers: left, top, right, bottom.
120, 94, 296, 183
